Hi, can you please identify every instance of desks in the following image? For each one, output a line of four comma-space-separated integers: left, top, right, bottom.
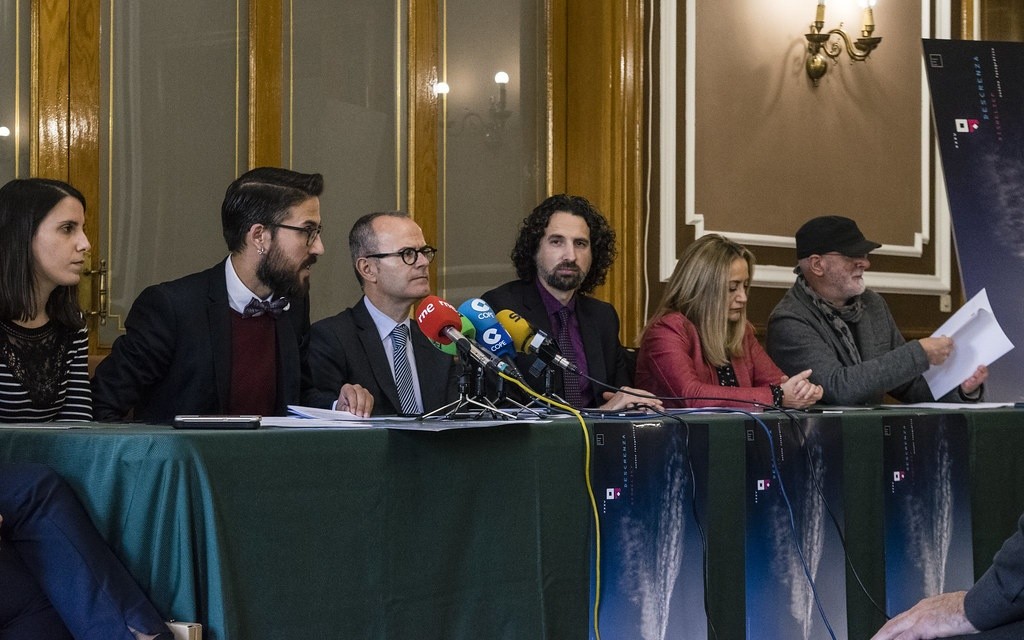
0, 403, 1024, 640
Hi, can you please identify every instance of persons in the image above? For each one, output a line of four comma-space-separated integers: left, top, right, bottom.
870, 513, 1024, 640
0, 177, 94, 424
633, 233, 823, 409
765, 215, 989, 405
311, 212, 477, 416
0, 466, 170, 640
480, 193, 665, 414
90, 167, 374, 425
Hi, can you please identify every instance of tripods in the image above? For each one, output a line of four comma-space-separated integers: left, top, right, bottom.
415, 338, 588, 421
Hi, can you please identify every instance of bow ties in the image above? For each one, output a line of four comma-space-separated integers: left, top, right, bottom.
241, 297, 290, 319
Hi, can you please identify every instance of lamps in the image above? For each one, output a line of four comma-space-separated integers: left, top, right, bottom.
804, 0, 884, 89
434, 72, 513, 154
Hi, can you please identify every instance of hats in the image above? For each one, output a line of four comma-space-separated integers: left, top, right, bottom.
795, 215, 883, 256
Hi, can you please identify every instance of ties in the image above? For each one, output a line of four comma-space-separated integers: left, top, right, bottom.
388, 325, 419, 415
554, 306, 583, 409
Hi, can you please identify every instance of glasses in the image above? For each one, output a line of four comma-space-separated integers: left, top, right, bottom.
272, 221, 323, 247
368, 246, 438, 265
824, 250, 869, 259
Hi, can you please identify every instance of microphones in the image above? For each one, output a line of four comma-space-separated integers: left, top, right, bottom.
415, 296, 583, 396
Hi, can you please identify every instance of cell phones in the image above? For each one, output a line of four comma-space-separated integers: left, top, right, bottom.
173, 415, 262, 429
